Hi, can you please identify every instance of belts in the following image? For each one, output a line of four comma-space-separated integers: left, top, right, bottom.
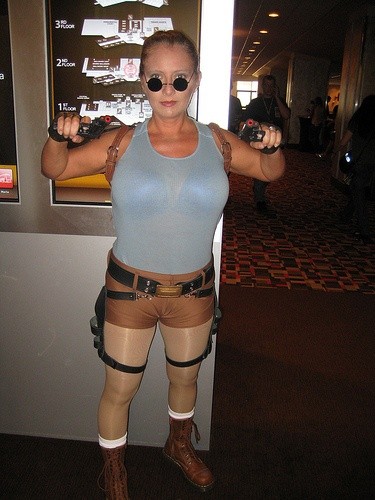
105, 256, 216, 301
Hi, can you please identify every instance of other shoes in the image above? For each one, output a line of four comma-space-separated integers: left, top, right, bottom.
256, 202, 267, 213
317, 152, 328, 160
339, 212, 348, 222
353, 230, 370, 241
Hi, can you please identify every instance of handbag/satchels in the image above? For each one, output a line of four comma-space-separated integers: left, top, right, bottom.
338, 154, 356, 174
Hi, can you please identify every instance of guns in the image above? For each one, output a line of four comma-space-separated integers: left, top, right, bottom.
52, 115, 112, 139
237, 120, 265, 142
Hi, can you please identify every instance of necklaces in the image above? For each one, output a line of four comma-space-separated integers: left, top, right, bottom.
261, 96, 275, 116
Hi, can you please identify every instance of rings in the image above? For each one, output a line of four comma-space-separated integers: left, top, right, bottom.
269, 125, 276, 131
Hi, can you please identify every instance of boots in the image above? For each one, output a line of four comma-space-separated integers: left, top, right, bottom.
96, 443, 129, 500
160, 416, 215, 490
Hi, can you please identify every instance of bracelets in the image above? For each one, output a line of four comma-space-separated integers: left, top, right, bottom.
260, 147, 279, 155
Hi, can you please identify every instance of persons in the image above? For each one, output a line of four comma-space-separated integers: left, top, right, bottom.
40, 30, 286, 500
316, 96, 337, 160
238, 75, 292, 212
335, 94, 375, 241
229, 82, 242, 135
308, 96, 325, 154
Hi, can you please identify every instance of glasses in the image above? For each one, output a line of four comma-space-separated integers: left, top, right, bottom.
141, 67, 196, 93
